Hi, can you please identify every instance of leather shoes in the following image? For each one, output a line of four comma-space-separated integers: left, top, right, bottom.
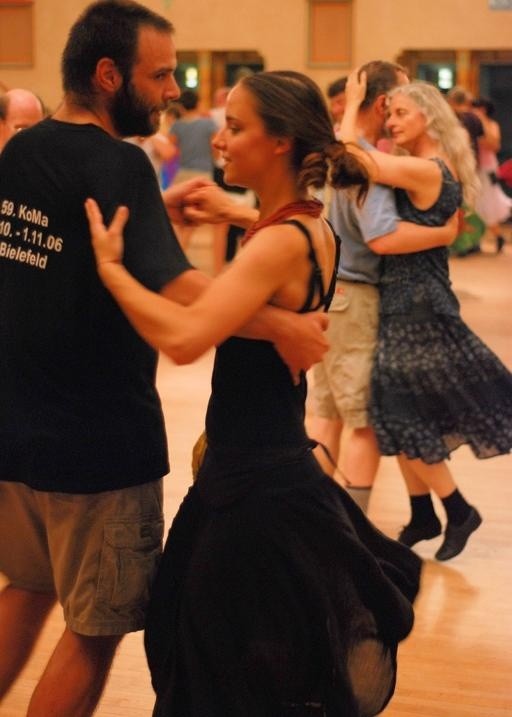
396, 514, 443, 548
435, 506, 482, 560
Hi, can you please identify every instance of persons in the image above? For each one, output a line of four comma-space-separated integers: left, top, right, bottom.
80, 67, 431, 717
327, 66, 512, 256
332, 66, 512, 564
0, 0, 336, 715
0, 79, 49, 163
135, 88, 257, 280
299, 57, 463, 522
